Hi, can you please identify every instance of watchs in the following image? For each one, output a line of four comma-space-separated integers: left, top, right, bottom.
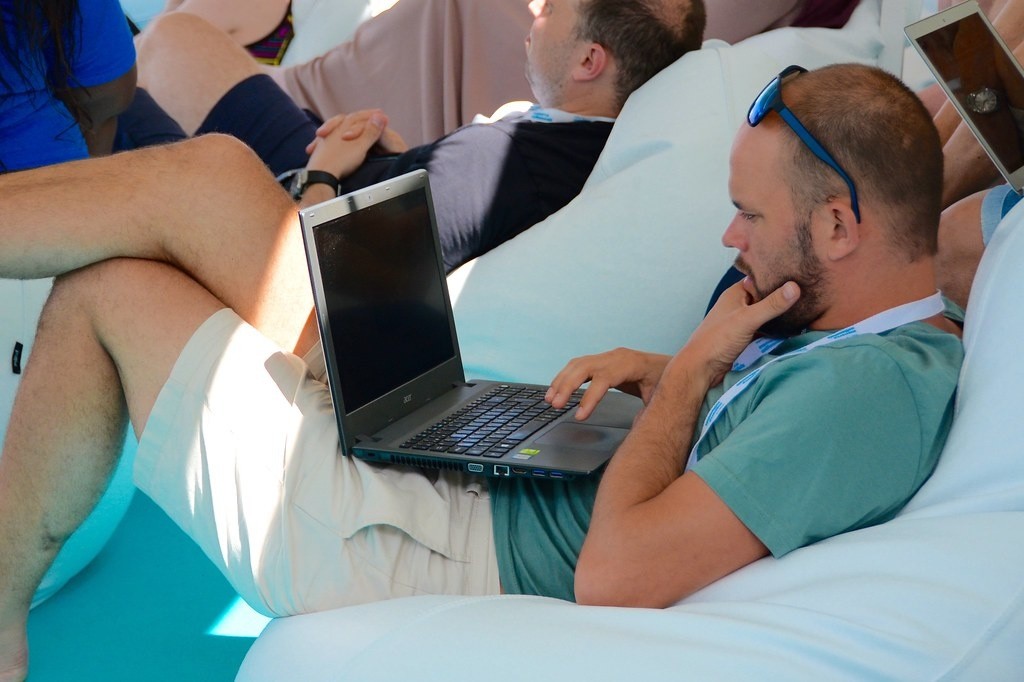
289, 167, 344, 200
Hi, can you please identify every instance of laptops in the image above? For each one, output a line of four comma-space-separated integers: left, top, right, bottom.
297, 169, 648, 482
901, 0, 1024, 195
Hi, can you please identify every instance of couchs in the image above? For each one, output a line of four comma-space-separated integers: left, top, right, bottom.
234, 201, 1024, 682
447, 24, 876, 382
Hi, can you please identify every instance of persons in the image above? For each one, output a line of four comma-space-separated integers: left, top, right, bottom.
0, 57, 969, 681
0, 0, 1024, 611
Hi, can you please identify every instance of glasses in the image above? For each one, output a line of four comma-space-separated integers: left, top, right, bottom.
748, 67, 863, 226
